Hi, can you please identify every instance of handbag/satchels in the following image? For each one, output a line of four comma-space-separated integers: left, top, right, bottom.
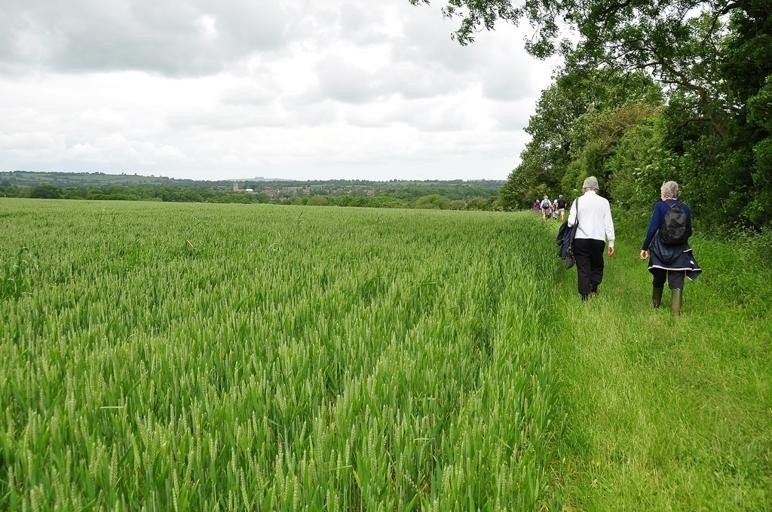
562, 223, 578, 256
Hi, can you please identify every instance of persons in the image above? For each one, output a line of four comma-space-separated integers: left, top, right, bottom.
566, 174, 617, 303
532, 194, 569, 222
639, 180, 703, 319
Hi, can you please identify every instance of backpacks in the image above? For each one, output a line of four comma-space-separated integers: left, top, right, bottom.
542, 200, 549, 208
660, 202, 687, 245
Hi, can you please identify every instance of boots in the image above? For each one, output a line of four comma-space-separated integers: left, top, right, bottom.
672, 288, 681, 315
653, 286, 663, 308
